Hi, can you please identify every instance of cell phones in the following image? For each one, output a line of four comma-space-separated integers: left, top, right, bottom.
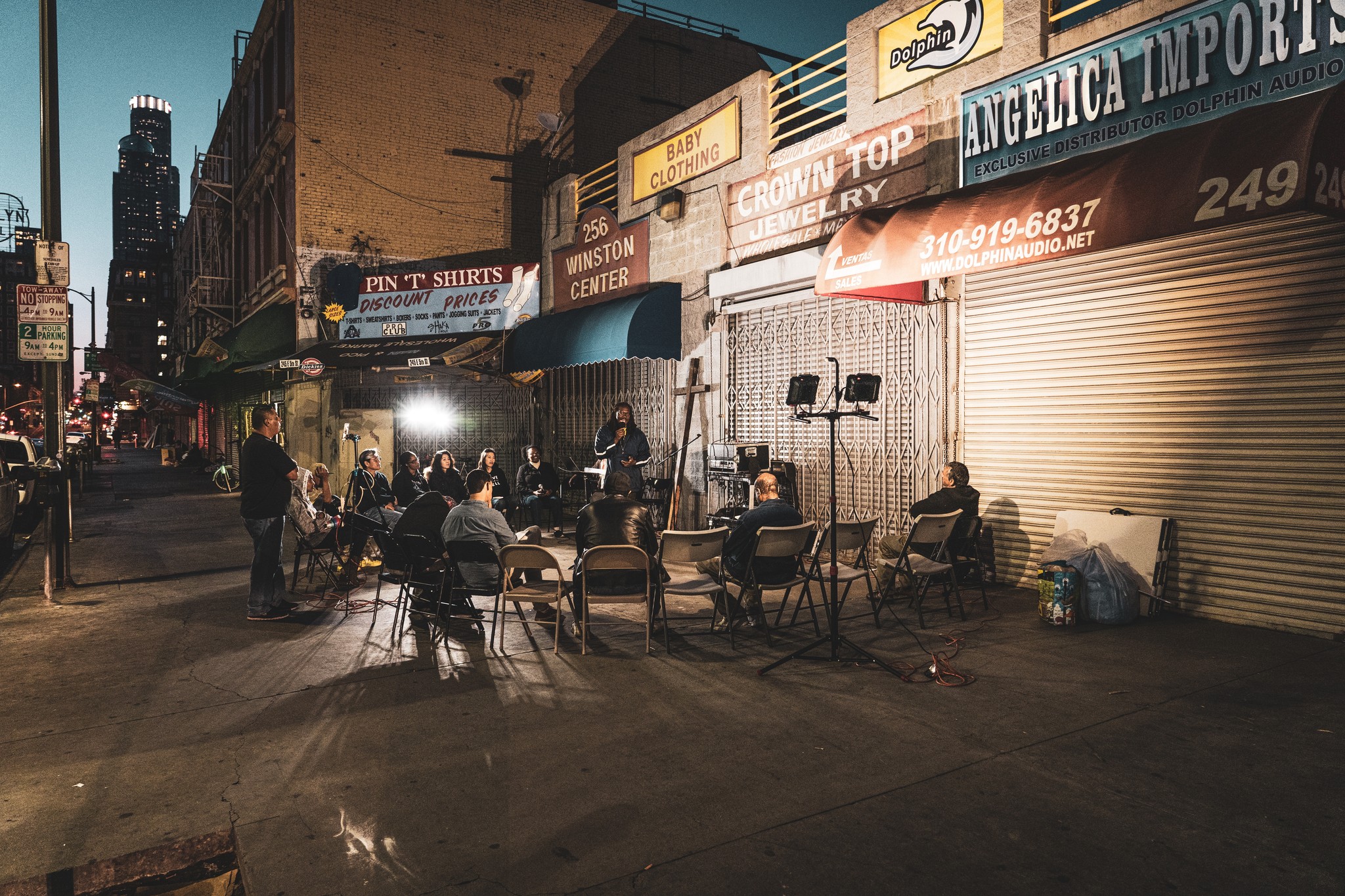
493, 496, 503, 499
341, 422, 349, 441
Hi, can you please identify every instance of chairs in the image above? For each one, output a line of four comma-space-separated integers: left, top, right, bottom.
494, 544, 583, 655
652, 521, 732, 651
871, 504, 966, 630
400, 530, 481, 641
441, 538, 533, 648
580, 543, 656, 657
912, 516, 985, 617
371, 528, 449, 632
791, 508, 884, 635
724, 516, 818, 643
644, 476, 674, 531
287, 510, 339, 590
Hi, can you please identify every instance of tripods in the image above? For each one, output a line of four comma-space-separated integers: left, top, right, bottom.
322, 434, 389, 616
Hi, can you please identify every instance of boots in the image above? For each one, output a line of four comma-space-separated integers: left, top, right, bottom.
339, 555, 365, 586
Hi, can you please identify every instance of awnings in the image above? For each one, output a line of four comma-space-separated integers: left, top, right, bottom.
501, 285, 682, 375
813, 81, 1345, 306
173, 299, 319, 390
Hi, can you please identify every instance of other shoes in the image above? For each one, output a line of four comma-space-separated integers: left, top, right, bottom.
570, 620, 582, 636
894, 586, 912, 596
277, 598, 299, 611
714, 613, 749, 631
408, 603, 430, 612
747, 613, 763, 626
554, 530, 565, 538
866, 589, 894, 604
450, 608, 483, 618
646, 617, 664, 630
248, 609, 289, 621
534, 606, 562, 621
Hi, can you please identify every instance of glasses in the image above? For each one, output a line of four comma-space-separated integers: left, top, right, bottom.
529, 451, 540, 455
617, 411, 630, 417
370, 458, 381, 462
408, 458, 419, 464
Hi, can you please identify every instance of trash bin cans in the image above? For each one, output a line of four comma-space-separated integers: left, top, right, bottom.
81, 445, 101, 462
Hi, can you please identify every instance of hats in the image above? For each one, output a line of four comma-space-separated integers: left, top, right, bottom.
603, 471, 631, 492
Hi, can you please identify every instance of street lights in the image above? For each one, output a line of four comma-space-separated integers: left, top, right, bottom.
4, 383, 20, 418
66, 287, 97, 482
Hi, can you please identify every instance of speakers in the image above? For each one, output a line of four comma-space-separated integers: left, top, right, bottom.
334, 262, 359, 311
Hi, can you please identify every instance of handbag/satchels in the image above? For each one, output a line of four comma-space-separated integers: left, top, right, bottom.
1037, 560, 1080, 628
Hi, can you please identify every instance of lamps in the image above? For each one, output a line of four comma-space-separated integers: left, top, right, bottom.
785, 374, 820, 415
845, 371, 884, 410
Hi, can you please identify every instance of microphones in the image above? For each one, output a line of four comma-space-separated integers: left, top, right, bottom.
617, 422, 626, 445
538, 484, 545, 491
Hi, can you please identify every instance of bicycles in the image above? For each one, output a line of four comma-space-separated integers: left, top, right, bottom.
212, 453, 240, 493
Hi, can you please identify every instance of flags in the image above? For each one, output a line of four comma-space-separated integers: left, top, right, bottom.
96, 351, 200, 419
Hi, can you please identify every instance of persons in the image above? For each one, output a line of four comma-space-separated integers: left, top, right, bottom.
439, 471, 561, 621
569, 459, 607, 503
384, 492, 485, 617
391, 451, 431, 508
594, 403, 651, 503
424, 450, 471, 506
353, 450, 407, 558
289, 467, 369, 587
175, 440, 203, 466
307, 464, 372, 557
477, 448, 517, 534
866, 462, 980, 603
516, 446, 562, 537
571, 470, 671, 640
695, 473, 803, 633
240, 406, 299, 620
112, 426, 122, 449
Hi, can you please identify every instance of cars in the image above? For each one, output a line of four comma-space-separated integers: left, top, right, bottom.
66, 432, 90, 445
71, 419, 81, 427
0, 433, 44, 535
